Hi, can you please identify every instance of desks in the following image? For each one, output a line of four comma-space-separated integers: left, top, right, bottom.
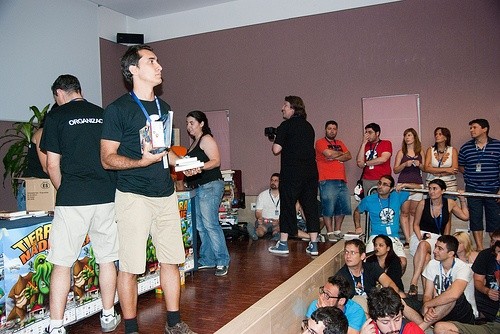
0, 190, 200, 334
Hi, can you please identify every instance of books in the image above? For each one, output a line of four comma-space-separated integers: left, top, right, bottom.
175, 157, 205, 172
219, 169, 238, 226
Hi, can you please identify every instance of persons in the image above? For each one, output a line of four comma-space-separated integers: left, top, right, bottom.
301, 275, 425, 334
434, 228, 500, 334
357, 123, 393, 197
181, 110, 230, 276
268, 96, 319, 255
100, 45, 198, 334
254, 173, 280, 237
295, 200, 325, 242
458, 119, 500, 252
408, 179, 469, 298
394, 128, 425, 250
38, 75, 121, 334
336, 234, 407, 299
425, 127, 458, 235
401, 236, 479, 334
353, 175, 422, 277
17, 103, 60, 211
315, 120, 352, 239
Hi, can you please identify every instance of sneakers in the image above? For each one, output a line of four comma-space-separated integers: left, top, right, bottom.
43, 325, 67, 334
306, 241, 319, 255
268, 240, 289, 254
215, 265, 229, 276
99, 309, 122, 333
197, 261, 216, 269
164, 320, 198, 334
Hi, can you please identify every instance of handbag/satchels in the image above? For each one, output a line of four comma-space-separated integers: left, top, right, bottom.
353, 180, 364, 202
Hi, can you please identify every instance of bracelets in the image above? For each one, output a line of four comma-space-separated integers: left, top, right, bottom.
460, 199, 466, 202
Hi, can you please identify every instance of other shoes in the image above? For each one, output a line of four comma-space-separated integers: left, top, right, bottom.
316, 234, 325, 243
325, 233, 337, 242
334, 232, 344, 239
246, 222, 259, 241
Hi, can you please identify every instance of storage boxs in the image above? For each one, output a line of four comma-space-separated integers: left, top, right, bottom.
14, 177, 57, 211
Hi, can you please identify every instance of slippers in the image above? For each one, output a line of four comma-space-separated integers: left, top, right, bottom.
408, 285, 418, 298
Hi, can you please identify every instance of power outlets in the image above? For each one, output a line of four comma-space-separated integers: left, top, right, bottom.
250, 203, 256, 211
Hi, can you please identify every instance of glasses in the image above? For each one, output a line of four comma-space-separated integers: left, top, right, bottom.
319, 286, 339, 301
377, 181, 391, 188
300, 319, 315, 334
